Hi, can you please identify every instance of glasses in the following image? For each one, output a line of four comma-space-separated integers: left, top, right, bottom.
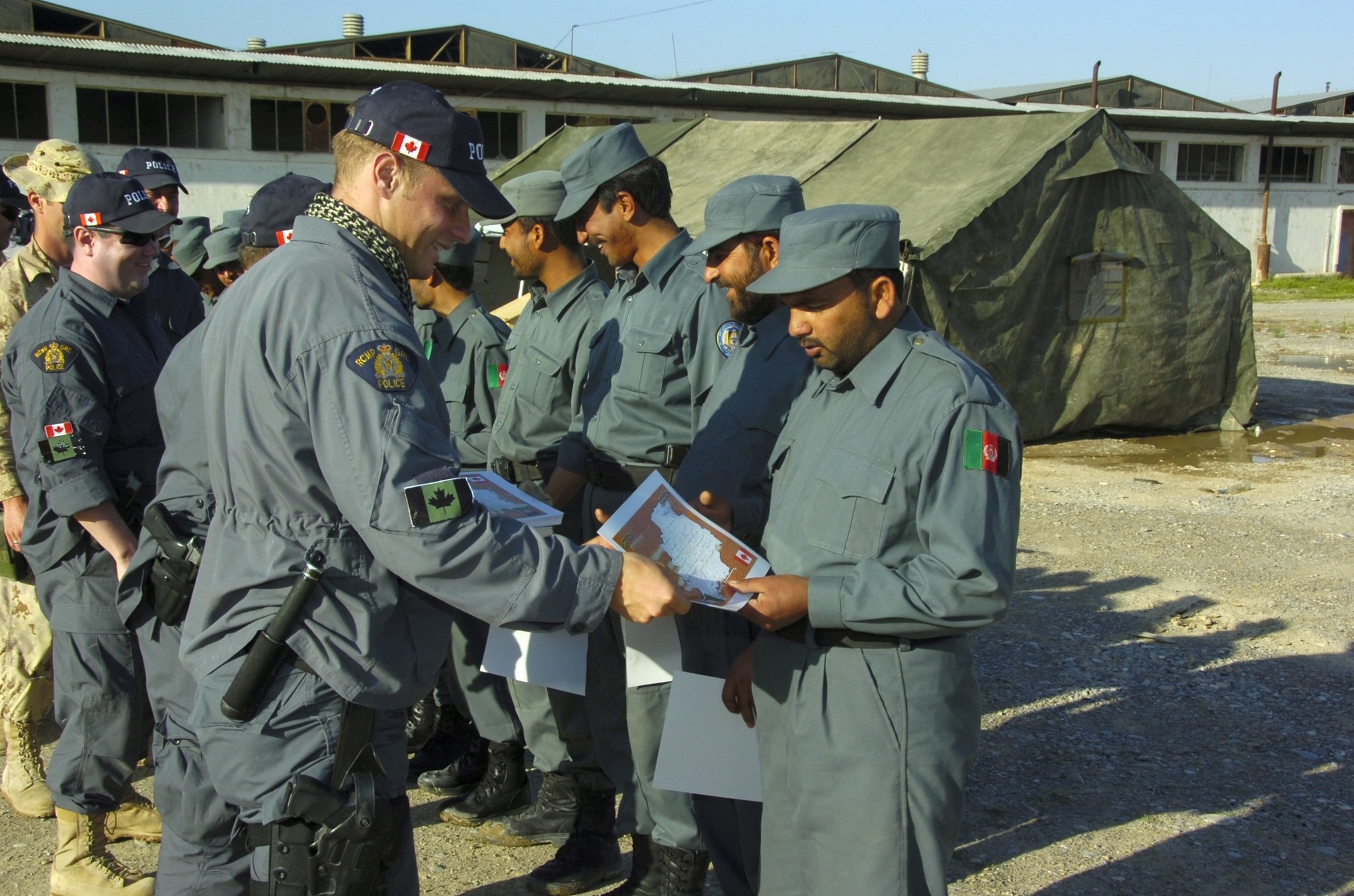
77, 226, 156, 247
0, 209, 21, 223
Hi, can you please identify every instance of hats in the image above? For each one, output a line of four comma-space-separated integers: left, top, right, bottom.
480, 170, 570, 227
203, 228, 242, 270
436, 225, 482, 267
345, 80, 517, 220
0, 139, 105, 209
212, 209, 247, 231
240, 172, 333, 248
158, 215, 211, 276
116, 148, 189, 196
680, 174, 806, 257
63, 171, 183, 237
746, 204, 901, 297
553, 122, 650, 222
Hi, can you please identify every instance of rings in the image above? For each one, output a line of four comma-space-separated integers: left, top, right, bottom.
13, 541, 19, 544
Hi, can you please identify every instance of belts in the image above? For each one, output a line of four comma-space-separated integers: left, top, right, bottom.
779, 620, 955, 649
585, 456, 677, 491
491, 456, 556, 482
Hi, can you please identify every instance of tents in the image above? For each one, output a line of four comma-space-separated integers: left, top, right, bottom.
470, 116, 1268, 441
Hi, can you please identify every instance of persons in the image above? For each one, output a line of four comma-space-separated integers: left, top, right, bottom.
725, 202, 1025, 896
123, 75, 703, 895
0, 170, 172, 896
0, 137, 331, 821
424, 118, 819, 896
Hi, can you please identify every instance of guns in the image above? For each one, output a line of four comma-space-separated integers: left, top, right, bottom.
140, 502, 205, 626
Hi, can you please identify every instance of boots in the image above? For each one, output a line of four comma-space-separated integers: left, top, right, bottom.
49, 807, 157, 896
406, 706, 465, 783
416, 732, 491, 795
599, 834, 662, 896
658, 846, 708, 896
527, 782, 624, 896
436, 741, 531, 827
479, 772, 579, 847
401, 699, 438, 753
101, 782, 163, 845
0, 719, 59, 818
0, 719, 8, 756
